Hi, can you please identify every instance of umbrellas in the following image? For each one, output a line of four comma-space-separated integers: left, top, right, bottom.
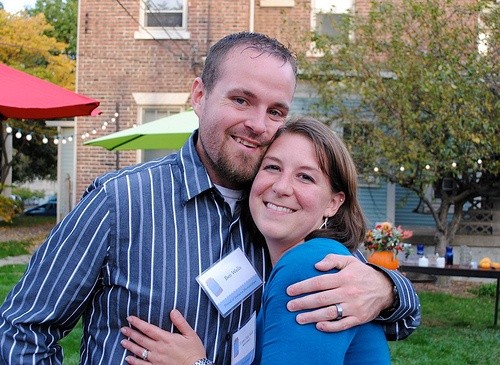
0, 59, 104, 122
84, 102, 208, 149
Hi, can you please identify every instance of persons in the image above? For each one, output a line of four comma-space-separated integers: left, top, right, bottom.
121, 115, 392, 365
0, 31, 422, 365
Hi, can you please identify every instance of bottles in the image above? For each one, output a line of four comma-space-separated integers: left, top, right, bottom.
445, 246, 453, 268
417, 244, 424, 258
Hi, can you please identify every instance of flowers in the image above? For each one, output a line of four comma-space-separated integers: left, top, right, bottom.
364, 221, 413, 261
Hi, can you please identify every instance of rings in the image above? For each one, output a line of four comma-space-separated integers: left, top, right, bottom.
142, 350, 150, 361
335, 305, 342, 320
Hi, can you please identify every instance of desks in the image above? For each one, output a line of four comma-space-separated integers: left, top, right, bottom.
352, 244, 500, 328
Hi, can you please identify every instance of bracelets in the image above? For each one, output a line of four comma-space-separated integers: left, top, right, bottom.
192, 358, 213, 365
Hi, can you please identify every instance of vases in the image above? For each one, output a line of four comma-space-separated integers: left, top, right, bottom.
367, 249, 399, 270
